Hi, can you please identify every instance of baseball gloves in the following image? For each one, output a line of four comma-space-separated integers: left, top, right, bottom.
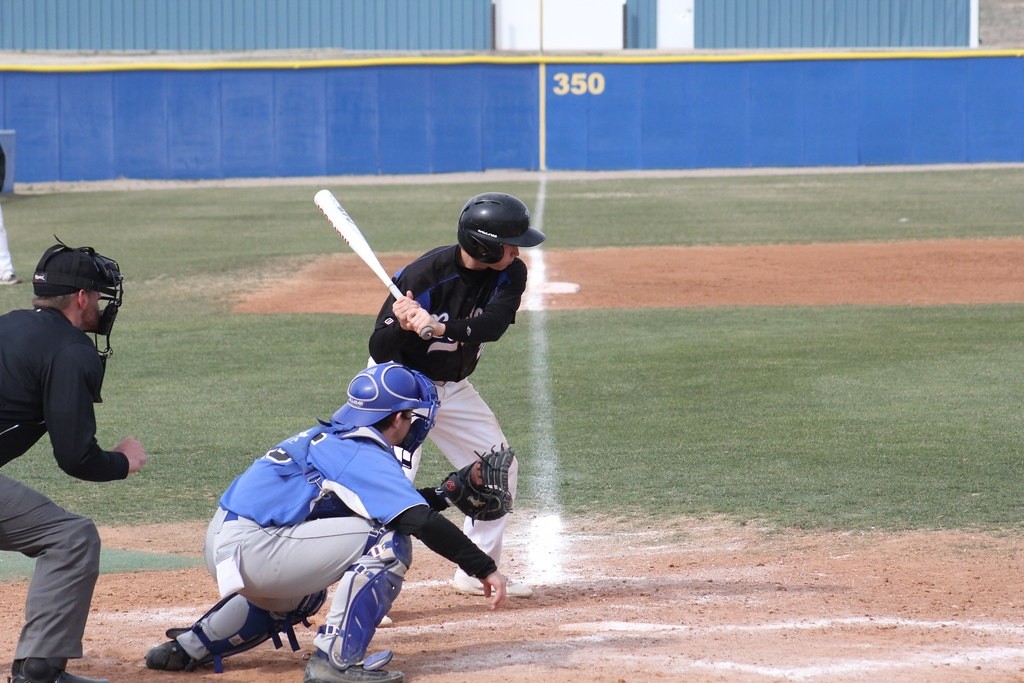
440, 449, 516, 521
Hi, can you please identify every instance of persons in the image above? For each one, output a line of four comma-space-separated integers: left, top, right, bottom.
0, 235, 147, 683
366, 193, 546, 627
144, 361, 514, 683
0, 144, 23, 285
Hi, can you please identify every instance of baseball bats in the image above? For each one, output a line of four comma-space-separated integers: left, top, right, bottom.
314, 188, 435, 340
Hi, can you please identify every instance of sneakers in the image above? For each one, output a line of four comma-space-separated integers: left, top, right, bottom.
454, 568, 534, 598
303, 652, 404, 683
378, 615, 393, 627
146, 640, 191, 671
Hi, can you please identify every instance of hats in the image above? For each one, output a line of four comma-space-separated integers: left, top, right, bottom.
33, 250, 119, 296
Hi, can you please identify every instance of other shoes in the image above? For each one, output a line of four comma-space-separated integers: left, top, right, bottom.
0, 275, 22, 285
12, 670, 109, 683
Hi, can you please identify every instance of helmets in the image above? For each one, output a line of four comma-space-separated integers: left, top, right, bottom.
333, 363, 418, 427
457, 192, 546, 264
